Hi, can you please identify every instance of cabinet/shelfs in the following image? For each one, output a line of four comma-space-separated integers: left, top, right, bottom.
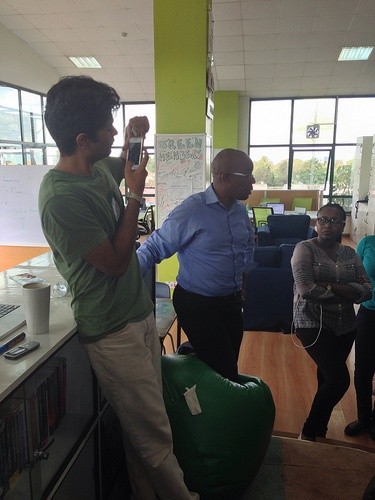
0, 329, 131, 500
349, 136, 375, 243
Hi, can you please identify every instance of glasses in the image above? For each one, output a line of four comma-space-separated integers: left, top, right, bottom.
316, 216, 346, 224
232, 172, 253, 182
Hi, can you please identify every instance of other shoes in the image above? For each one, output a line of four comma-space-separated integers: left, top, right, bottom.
368, 417, 375, 442
345, 420, 366, 437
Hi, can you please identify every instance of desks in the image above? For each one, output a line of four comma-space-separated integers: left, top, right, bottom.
156, 298, 181, 355
248, 209, 318, 219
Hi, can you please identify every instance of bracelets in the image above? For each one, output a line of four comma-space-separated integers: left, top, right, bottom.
125, 191, 145, 207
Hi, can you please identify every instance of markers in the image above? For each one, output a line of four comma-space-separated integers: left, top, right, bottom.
0, 331, 26, 356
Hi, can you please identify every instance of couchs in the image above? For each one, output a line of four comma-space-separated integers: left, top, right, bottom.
243, 215, 318, 330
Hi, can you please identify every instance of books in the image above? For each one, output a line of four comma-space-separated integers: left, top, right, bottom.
0, 357, 67, 500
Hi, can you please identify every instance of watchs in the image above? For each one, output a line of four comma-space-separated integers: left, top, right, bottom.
327, 281, 333, 290
239, 294, 248, 301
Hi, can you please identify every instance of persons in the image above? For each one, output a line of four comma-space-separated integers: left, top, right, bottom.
291, 203, 373, 441
39, 75, 200, 500
135, 148, 256, 383
344, 235, 375, 441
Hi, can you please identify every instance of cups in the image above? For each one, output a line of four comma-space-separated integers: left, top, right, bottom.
23, 282, 51, 335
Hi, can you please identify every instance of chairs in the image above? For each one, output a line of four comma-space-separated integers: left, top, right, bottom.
252, 197, 312, 228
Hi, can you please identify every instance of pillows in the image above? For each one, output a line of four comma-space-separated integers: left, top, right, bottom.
280, 244, 295, 268
254, 246, 279, 267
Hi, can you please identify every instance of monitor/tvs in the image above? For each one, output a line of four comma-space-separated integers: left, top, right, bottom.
266, 203, 285, 215
252, 206, 274, 228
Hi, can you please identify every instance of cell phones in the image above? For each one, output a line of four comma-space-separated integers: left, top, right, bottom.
126, 137, 143, 170
4, 340, 40, 360
